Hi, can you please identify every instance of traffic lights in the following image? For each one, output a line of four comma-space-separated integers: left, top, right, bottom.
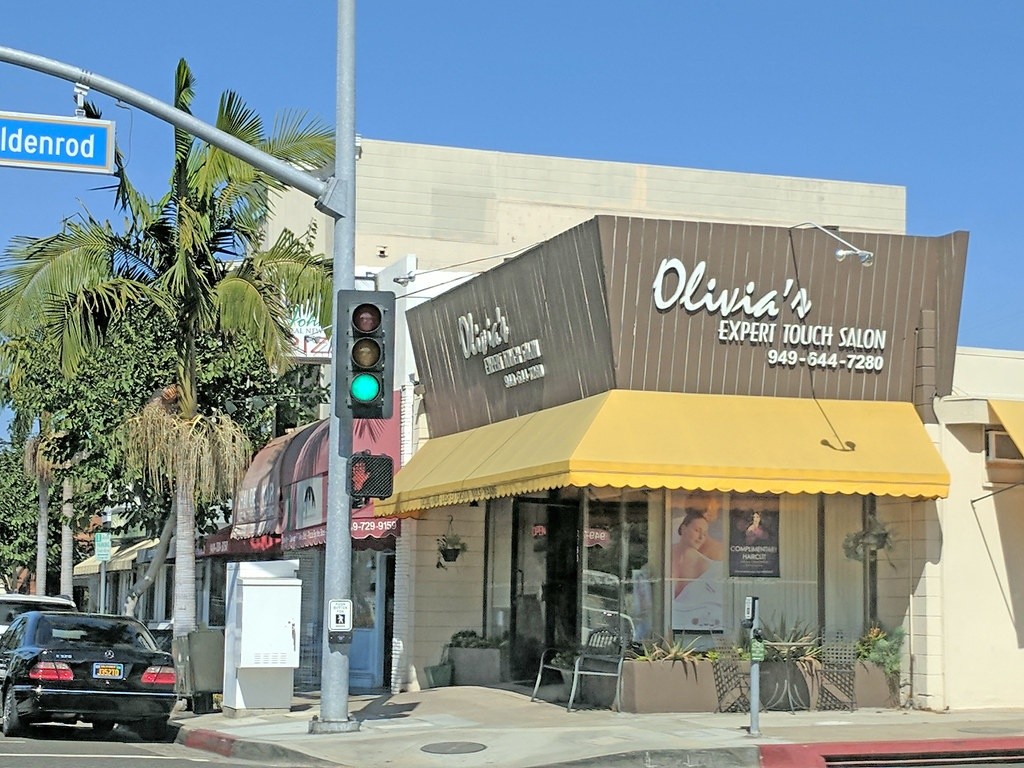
333, 289, 397, 421
349, 452, 395, 500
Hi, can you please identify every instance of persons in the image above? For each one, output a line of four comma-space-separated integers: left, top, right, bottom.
672, 494, 722, 600
745, 510, 767, 540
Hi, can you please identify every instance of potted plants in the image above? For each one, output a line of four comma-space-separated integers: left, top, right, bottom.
435, 533, 466, 570
620, 623, 904, 714
841, 520, 904, 571
447, 631, 512, 689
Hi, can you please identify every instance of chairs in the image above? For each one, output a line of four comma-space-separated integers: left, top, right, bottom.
531, 627, 625, 711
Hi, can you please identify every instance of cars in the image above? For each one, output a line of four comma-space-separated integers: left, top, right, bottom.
0, 609, 179, 740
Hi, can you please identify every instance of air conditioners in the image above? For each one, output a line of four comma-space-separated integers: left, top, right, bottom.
984, 430, 1024, 464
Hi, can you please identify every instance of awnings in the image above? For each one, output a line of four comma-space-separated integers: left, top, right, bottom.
230, 419, 322, 541
73, 538, 160, 575
986, 398, 1024, 460
372, 389, 952, 520
280, 389, 401, 552
203, 524, 282, 557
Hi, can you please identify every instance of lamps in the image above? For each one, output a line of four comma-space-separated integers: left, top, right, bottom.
789, 221, 875, 269
393, 238, 541, 287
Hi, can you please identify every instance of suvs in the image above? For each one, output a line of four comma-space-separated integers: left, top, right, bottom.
0, 592, 76, 644
